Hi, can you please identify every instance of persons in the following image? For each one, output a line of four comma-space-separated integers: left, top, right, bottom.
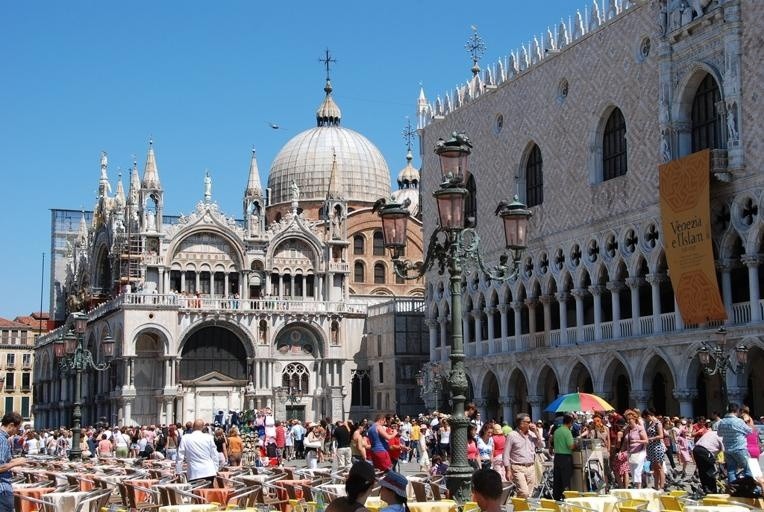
258, 293, 289, 312
133, 287, 200, 307
221, 292, 239, 309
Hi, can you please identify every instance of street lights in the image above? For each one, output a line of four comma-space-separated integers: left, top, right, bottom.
276, 378, 303, 422
697, 327, 750, 419
415, 360, 453, 413
371, 132, 533, 512
52, 310, 116, 462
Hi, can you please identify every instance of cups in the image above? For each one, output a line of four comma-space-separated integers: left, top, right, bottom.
573, 438, 602, 452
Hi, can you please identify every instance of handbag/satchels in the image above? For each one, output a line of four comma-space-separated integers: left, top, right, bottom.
303, 433, 322, 448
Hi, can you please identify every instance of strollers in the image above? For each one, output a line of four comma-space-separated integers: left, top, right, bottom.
585, 458, 602, 492
536, 451, 554, 498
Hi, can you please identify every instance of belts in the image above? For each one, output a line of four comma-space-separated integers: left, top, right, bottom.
512, 462, 534, 467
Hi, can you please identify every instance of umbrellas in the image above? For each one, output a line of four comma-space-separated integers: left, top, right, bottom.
541, 387, 616, 431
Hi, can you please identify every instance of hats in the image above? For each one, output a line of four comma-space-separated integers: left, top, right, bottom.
654, 415, 713, 427
307, 422, 318, 429
376, 471, 409, 500
493, 424, 503, 435
537, 419, 542, 423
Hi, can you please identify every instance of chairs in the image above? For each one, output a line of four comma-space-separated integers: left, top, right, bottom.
0, 456, 762, 511
